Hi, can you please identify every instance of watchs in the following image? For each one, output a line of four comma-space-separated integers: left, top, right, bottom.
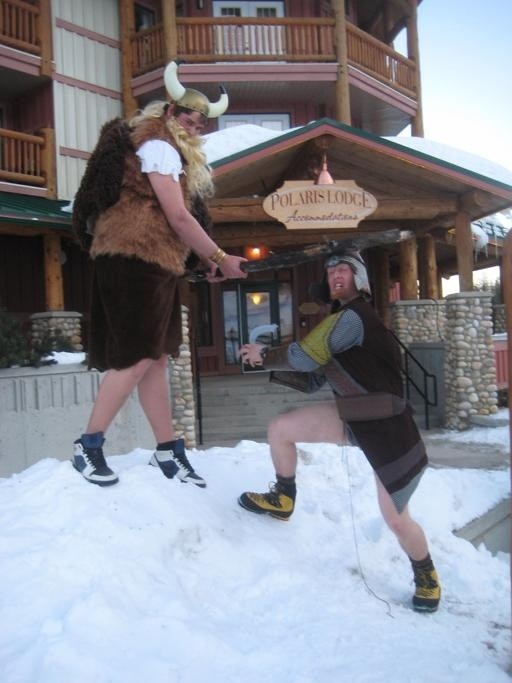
260, 346, 268, 359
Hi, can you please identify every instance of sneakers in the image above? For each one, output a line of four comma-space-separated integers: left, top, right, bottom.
70, 429, 119, 487
234, 484, 298, 522
148, 436, 209, 491
409, 564, 444, 614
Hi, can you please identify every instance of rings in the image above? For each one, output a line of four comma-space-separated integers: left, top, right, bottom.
246, 348, 250, 352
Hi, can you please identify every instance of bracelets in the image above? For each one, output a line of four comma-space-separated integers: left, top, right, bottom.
208, 246, 227, 265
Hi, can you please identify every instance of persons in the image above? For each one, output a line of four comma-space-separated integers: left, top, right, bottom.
236, 247, 440, 612
70, 57, 249, 488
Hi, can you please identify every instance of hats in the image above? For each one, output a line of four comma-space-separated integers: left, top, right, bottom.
159, 57, 231, 121
321, 237, 374, 298
